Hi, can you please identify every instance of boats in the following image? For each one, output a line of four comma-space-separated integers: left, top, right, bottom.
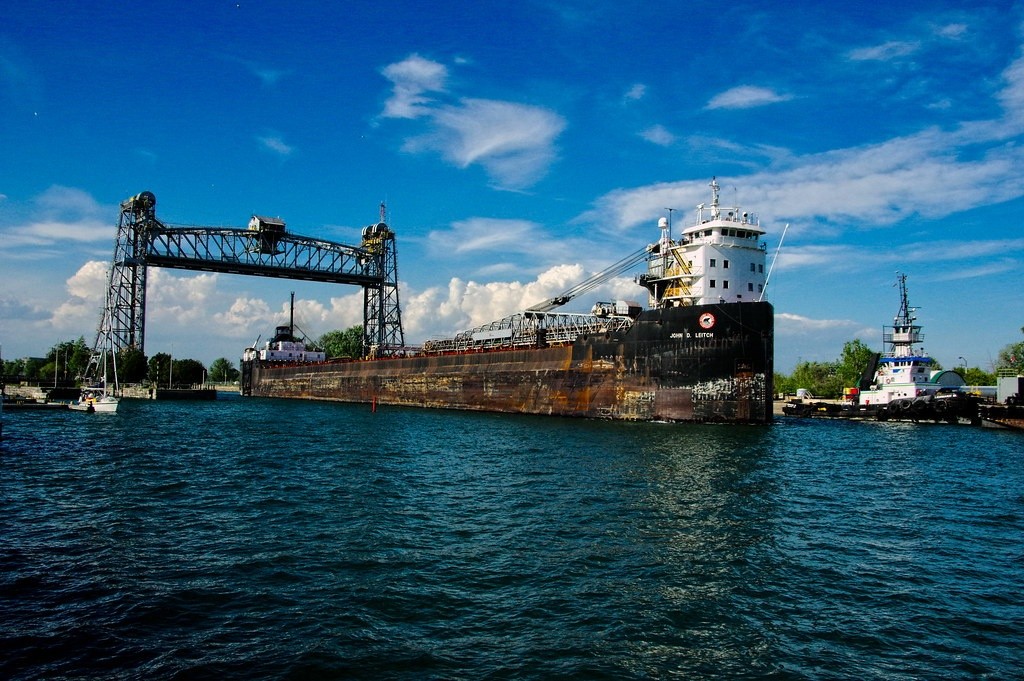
237, 173, 790, 431
779, 268, 984, 428
983, 401, 1024, 431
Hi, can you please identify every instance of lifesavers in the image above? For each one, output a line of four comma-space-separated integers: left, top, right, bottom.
886, 378, 890, 383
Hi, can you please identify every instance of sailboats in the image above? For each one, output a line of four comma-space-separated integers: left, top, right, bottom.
67, 270, 119, 413
